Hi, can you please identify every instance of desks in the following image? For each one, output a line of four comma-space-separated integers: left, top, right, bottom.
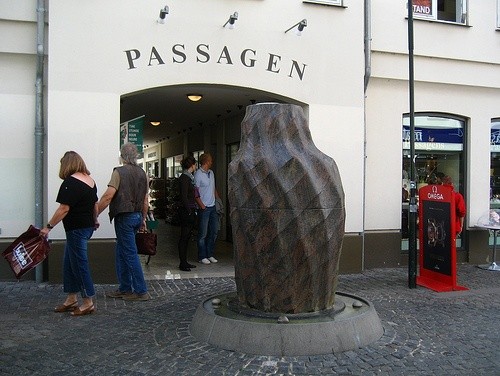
474, 223, 500, 270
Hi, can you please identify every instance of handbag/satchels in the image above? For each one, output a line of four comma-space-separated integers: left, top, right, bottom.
135, 229, 156, 255
2, 225, 52, 279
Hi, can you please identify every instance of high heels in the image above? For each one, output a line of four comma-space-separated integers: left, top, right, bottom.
55, 300, 79, 312
71, 304, 95, 316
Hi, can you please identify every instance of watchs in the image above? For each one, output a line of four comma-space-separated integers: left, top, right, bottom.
47, 223, 53, 229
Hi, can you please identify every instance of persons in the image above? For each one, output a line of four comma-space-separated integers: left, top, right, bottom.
441, 176, 466, 235
176, 156, 198, 271
194, 154, 220, 264
40, 151, 98, 316
98, 142, 149, 301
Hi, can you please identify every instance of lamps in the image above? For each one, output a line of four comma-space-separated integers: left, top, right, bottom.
187, 95, 202, 102
222, 12, 238, 30
285, 19, 307, 36
157, 5, 168, 24
150, 121, 160, 126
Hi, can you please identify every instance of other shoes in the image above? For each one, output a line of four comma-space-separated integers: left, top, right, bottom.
200, 258, 211, 265
123, 291, 148, 301
186, 264, 197, 268
179, 267, 191, 272
109, 290, 132, 298
208, 257, 217, 262
